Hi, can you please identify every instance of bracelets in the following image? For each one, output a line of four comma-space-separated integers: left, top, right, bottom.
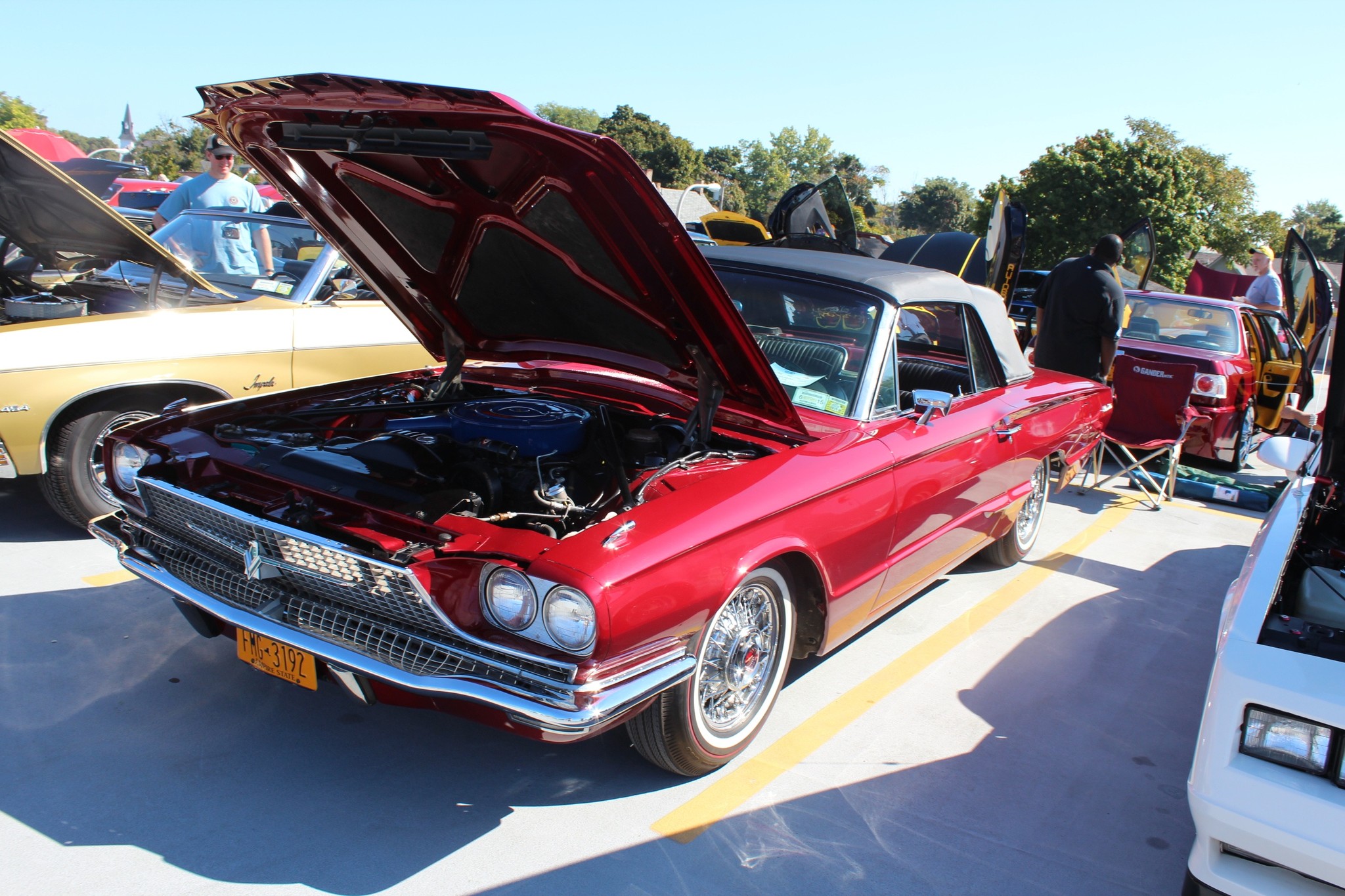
264, 268, 275, 272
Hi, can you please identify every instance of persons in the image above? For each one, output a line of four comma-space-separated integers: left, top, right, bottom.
152, 134, 276, 278
1281, 406, 1325, 432
1032, 233, 1126, 478
158, 174, 170, 182
1239, 245, 1283, 336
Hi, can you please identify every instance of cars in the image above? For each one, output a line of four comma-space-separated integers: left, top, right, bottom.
1184, 264, 1345, 896
89, 74, 1114, 787
5, 126, 287, 237
1109, 226, 1339, 475
700, 212, 769, 246
0, 132, 448, 534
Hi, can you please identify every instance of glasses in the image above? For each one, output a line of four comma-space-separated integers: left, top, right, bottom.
214, 155, 234, 160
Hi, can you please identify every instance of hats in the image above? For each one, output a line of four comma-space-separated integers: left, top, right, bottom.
205, 134, 238, 155
156, 166, 164, 171
1249, 245, 1274, 261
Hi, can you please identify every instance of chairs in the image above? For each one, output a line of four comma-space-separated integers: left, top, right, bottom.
1077, 354, 1212, 511
745, 340, 846, 411
1183, 327, 1230, 360
1125, 315, 1171, 349
876, 358, 990, 414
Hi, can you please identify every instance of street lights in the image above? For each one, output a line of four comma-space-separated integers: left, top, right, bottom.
673, 183, 721, 219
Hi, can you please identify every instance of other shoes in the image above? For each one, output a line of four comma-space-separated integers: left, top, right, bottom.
1274, 479, 1290, 489
